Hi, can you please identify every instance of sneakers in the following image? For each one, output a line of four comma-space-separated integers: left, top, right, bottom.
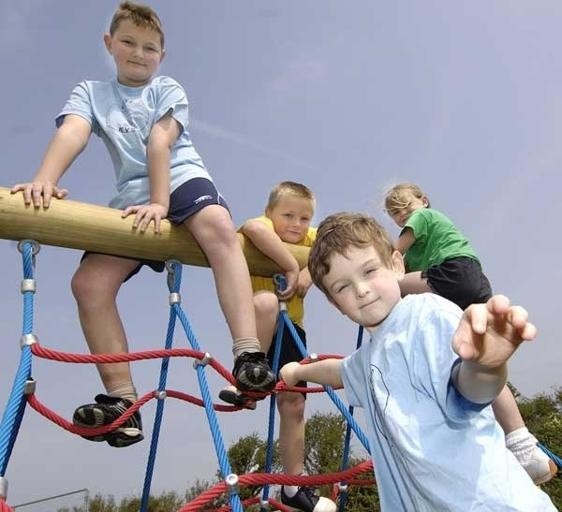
72, 393, 144, 448
233, 351, 276, 392
218, 384, 256, 411
522, 439, 562, 486
279, 484, 337, 512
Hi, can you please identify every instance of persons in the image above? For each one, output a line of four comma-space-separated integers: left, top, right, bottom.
273, 209, 562, 511
228, 178, 342, 511
9, 1, 276, 451
376, 179, 561, 491
